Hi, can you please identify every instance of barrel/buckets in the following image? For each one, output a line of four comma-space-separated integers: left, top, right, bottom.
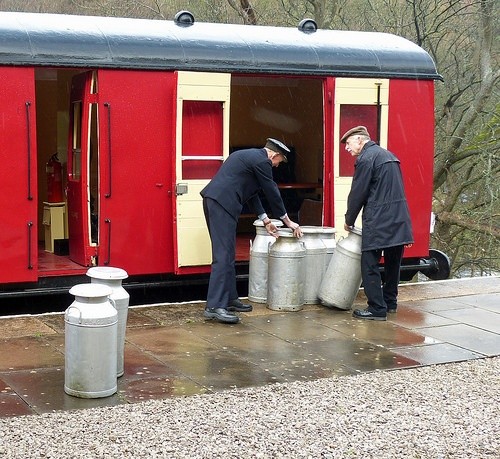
246, 218, 361, 313
63, 266, 130, 399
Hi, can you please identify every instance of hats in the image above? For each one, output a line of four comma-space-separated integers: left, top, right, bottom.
265, 138, 290, 160
340, 126, 370, 143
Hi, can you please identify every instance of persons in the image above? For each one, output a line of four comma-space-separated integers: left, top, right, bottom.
200, 138, 302, 324
340, 125, 414, 321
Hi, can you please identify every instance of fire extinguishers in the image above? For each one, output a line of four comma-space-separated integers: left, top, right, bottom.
46, 152, 64, 203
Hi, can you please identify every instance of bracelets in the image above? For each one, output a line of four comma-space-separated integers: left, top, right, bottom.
263, 220, 270, 225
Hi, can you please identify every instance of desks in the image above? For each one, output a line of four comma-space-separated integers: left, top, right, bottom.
42, 202, 69, 254
275, 183, 324, 216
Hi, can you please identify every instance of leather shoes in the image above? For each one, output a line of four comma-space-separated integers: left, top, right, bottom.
205, 307, 239, 323
226, 300, 253, 312
352, 307, 387, 321
388, 308, 397, 312
